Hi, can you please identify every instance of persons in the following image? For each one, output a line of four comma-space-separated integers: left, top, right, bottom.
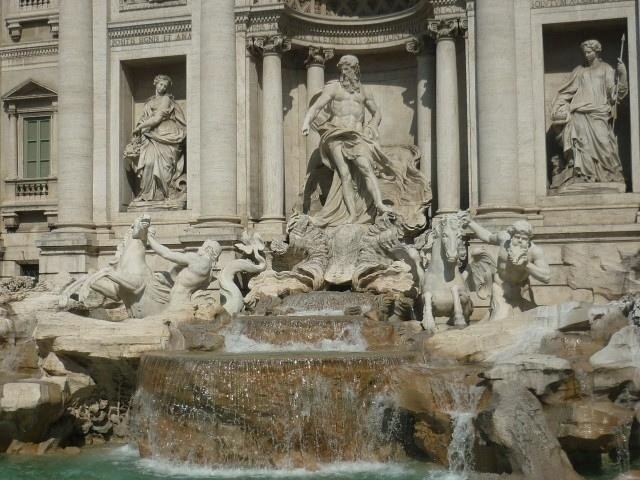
300, 53, 392, 224
549, 40, 627, 192
458, 210, 552, 320
123, 73, 187, 204
144, 225, 221, 303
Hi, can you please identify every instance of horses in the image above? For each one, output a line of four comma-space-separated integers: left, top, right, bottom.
380, 212, 495, 330
89, 215, 173, 318
